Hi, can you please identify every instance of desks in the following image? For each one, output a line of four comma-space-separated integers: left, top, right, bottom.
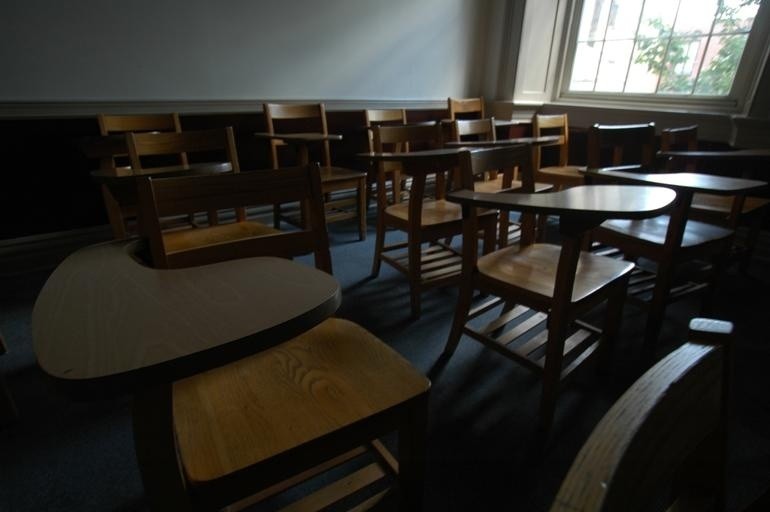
30, 238, 340, 391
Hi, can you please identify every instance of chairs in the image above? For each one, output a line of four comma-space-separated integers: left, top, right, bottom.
546, 317, 755, 511
102, 97, 369, 271
171, 317, 433, 512
369, 99, 760, 435
